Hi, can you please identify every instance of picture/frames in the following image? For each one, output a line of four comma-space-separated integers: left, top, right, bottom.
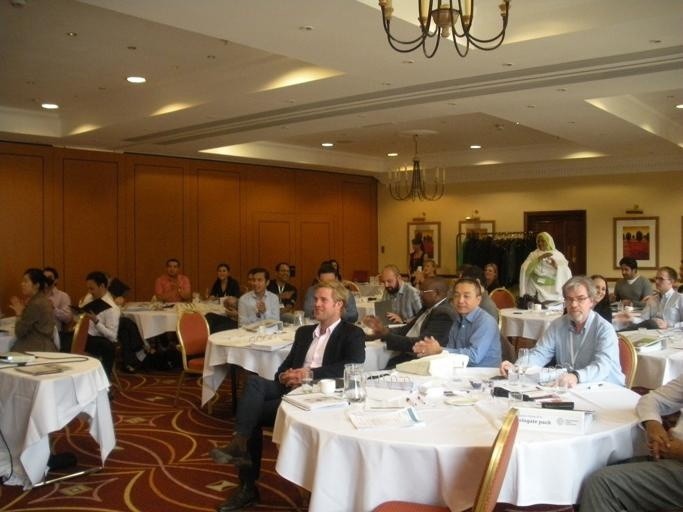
407, 221, 441, 268
613, 216, 659, 270
458, 220, 496, 271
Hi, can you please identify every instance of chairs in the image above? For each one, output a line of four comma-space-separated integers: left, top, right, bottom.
371, 406, 523, 511
69, 313, 90, 354
489, 286, 515, 309
174, 306, 238, 415
351, 270, 369, 282
617, 334, 639, 388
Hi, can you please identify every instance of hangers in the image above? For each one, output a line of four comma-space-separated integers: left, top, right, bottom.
462, 231, 537, 241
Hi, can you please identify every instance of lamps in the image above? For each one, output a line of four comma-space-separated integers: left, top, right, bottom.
378, 0, 513, 58
387, 135, 446, 201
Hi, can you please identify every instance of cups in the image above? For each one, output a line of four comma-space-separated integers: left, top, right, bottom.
319, 379, 335, 394
528, 301, 541, 312
191, 292, 199, 304
344, 348, 571, 410
300, 370, 313, 394
638, 328, 647, 338
617, 302, 633, 312
248, 310, 305, 345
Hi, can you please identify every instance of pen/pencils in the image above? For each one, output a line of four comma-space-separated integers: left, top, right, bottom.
417, 396, 426, 405
406, 398, 415, 406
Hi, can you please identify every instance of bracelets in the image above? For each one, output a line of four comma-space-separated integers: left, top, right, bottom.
570, 370, 581, 384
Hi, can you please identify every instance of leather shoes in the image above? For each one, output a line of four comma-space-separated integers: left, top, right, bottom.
216, 485, 259, 511
211, 445, 252, 467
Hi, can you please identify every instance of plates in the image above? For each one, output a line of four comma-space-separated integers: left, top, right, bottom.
0, 355, 34, 364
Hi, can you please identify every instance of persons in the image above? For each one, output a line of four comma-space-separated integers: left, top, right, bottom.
155, 258, 192, 348
365, 264, 502, 371
499, 278, 625, 389
678, 259, 683, 293
616, 266, 683, 329
564, 275, 612, 323
10, 268, 152, 384
580, 371, 683, 510
519, 231, 573, 313
610, 257, 653, 307
406, 238, 429, 287
305, 260, 359, 324
210, 263, 297, 330
207, 278, 366, 512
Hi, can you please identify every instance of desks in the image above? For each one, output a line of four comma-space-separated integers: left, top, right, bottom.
272, 365, 649, 512
114, 301, 286, 346
618, 323, 683, 390
0, 281, 683, 511
199, 320, 407, 408
0, 349, 116, 492
498, 307, 640, 352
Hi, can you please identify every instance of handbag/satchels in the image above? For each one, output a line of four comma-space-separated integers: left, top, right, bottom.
516, 294, 545, 309
